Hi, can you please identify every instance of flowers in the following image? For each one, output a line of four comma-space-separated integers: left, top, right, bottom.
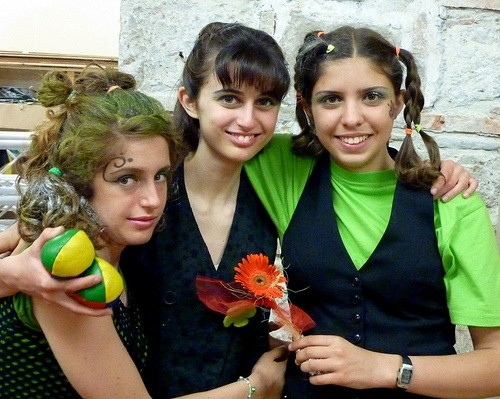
194, 253, 327, 377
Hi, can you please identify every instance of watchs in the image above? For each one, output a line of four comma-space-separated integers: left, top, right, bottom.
396, 356, 415, 394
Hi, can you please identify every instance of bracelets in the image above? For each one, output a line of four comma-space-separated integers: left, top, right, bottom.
235, 375, 256, 399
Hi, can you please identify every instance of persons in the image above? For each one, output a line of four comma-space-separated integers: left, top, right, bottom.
243, 26, 500, 399
0, 21, 479, 399
0, 62, 288, 399
